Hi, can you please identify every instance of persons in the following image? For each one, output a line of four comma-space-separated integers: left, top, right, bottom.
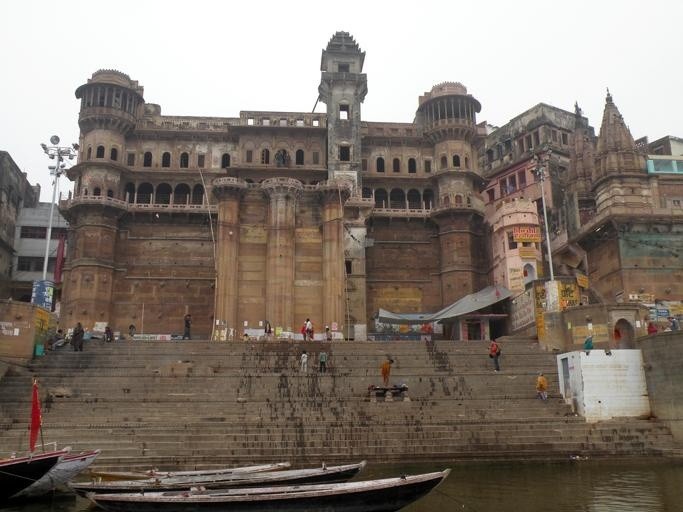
536, 372, 548, 401
379, 359, 394, 387
264, 322, 271, 336
70, 322, 84, 352
182, 314, 192, 340
300, 351, 307, 373
104, 326, 113, 343
487, 336, 500, 372
647, 322, 658, 334
667, 316, 681, 331
323, 318, 338, 340
128, 323, 136, 341
301, 318, 314, 342
584, 336, 593, 349
318, 348, 328, 373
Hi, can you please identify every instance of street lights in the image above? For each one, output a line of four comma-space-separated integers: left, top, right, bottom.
40, 136, 79, 281
526, 142, 554, 280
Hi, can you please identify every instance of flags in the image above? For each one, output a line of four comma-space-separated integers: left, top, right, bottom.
30, 383, 41, 456
53, 238, 64, 284
